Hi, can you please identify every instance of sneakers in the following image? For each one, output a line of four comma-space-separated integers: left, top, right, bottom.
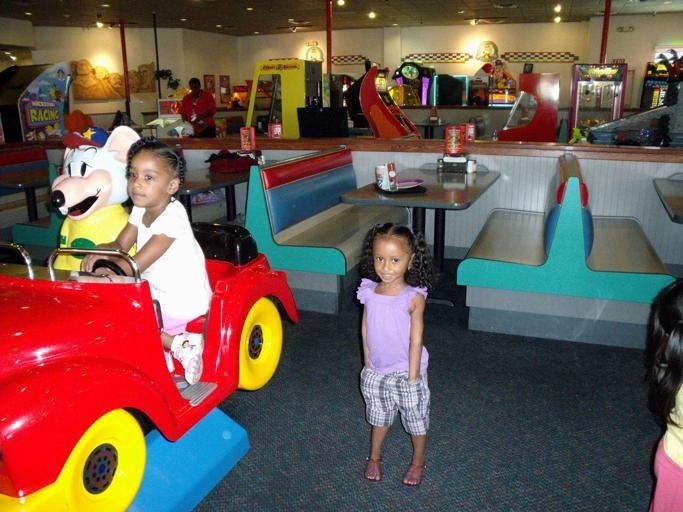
169, 332, 204, 385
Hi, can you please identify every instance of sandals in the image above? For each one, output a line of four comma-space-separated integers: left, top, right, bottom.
365, 456, 382, 481
402, 465, 426, 485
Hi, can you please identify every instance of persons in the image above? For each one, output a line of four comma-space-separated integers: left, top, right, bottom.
84, 136, 215, 385
181, 78, 216, 139
354, 222, 431, 486
643, 278, 682, 512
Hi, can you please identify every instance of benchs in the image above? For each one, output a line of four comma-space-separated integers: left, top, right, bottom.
244, 145, 411, 315
12, 162, 64, 261
569, 153, 676, 350
456, 152, 569, 341
0, 144, 51, 230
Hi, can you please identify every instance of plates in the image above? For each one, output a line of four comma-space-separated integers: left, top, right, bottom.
397, 178, 425, 189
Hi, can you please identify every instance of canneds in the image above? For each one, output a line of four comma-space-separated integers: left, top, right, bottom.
444, 125, 463, 154
374, 164, 389, 190
384, 162, 397, 190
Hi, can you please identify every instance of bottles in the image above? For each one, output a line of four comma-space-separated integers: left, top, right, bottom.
240, 116, 282, 165
215, 117, 227, 140
375, 160, 397, 191
437, 120, 476, 173
